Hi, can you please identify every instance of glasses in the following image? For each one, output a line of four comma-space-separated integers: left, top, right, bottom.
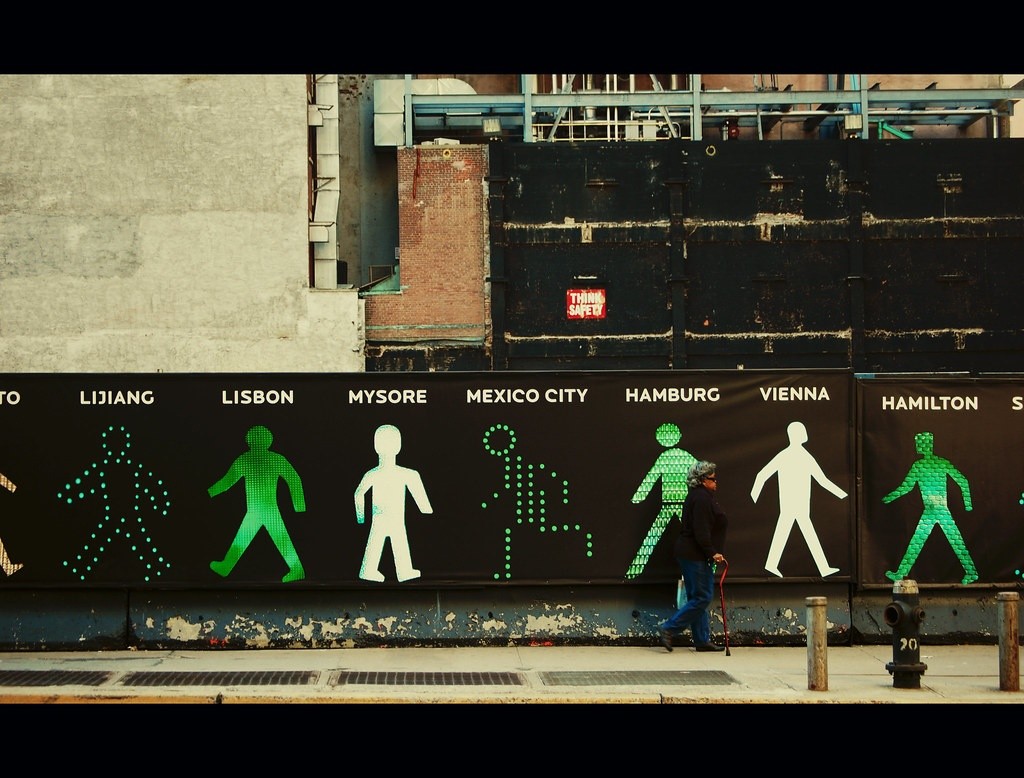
703, 477, 716, 483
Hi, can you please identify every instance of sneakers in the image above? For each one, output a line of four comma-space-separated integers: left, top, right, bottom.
659, 624, 673, 652
697, 642, 724, 652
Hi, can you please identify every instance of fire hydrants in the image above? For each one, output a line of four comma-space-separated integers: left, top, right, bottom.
883, 579, 928, 689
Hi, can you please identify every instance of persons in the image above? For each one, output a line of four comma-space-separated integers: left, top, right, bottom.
655, 462, 731, 654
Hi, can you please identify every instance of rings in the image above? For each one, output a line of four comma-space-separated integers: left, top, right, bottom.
717, 559, 720, 562
